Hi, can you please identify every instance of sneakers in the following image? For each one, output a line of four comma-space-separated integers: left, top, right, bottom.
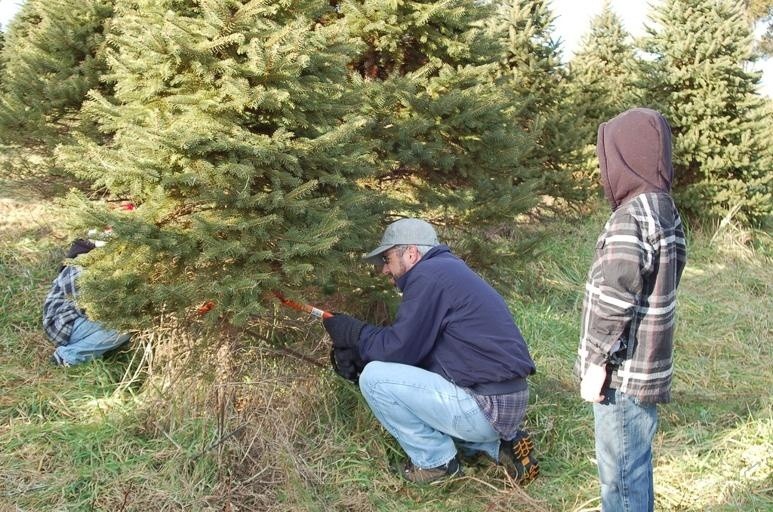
498, 429, 541, 488
400, 460, 462, 487
51, 352, 69, 368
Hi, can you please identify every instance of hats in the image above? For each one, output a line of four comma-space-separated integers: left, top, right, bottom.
363, 218, 439, 259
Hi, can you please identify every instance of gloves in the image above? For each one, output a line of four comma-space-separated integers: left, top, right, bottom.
333, 345, 365, 381
325, 312, 365, 349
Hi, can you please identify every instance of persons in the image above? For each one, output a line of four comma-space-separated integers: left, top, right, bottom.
42, 238, 131, 369
323, 216, 540, 488
571, 108, 687, 512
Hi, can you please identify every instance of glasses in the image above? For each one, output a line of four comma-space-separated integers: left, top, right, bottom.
381, 255, 390, 264
